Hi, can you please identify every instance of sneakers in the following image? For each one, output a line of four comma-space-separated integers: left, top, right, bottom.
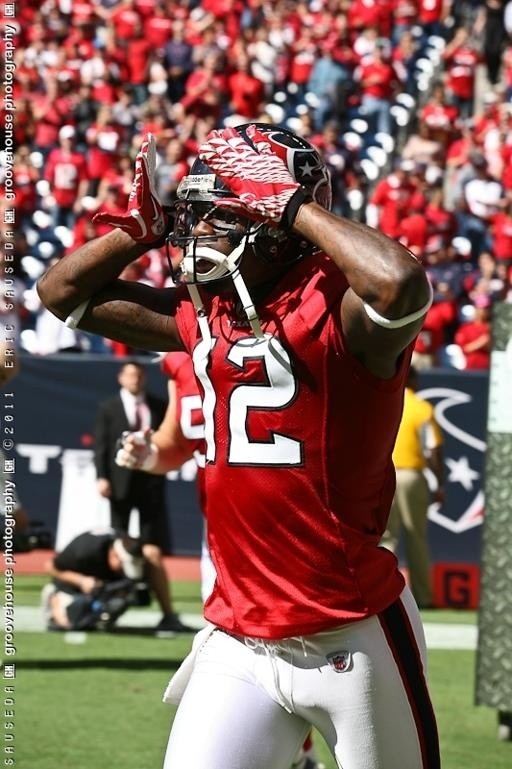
153, 612, 203, 639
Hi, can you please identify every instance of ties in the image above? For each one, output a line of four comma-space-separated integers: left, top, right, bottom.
133, 402, 144, 430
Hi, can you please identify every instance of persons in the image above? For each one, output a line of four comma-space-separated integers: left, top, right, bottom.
35, 123, 444, 769
92, 360, 202, 636
39, 525, 151, 633
115, 351, 325, 769
0, 0, 511, 381
378, 365, 442, 610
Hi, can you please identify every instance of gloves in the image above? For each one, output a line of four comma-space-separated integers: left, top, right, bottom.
89, 130, 172, 249
193, 120, 304, 228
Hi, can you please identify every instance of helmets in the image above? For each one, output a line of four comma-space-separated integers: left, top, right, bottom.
177, 122, 334, 267
